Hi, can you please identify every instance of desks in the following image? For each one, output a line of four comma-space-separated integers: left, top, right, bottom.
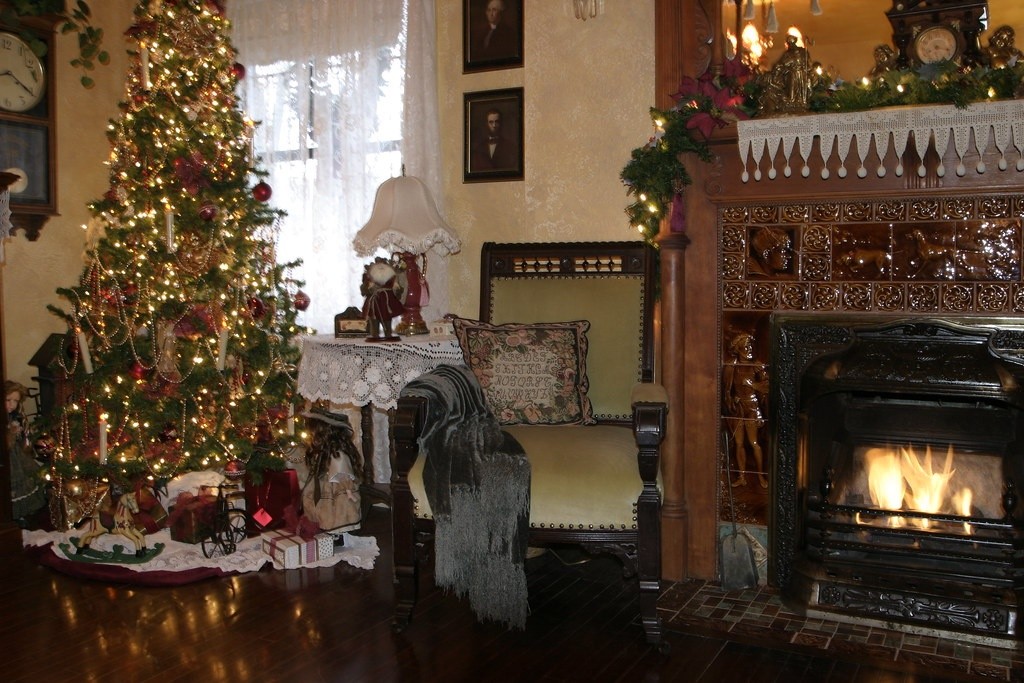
306, 332, 464, 533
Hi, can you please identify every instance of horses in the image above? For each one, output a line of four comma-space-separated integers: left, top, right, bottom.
74, 491, 147, 558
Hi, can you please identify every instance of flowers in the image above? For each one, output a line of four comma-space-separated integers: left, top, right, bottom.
617, 52, 1024, 242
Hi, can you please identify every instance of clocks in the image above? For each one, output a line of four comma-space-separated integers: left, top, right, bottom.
0, 5, 67, 216
887, 1, 988, 69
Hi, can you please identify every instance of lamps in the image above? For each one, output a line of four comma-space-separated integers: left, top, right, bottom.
351, 175, 460, 336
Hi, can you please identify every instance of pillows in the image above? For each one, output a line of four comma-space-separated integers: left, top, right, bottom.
451, 319, 595, 427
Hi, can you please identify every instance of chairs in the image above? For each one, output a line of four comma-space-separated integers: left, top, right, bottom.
388, 241, 663, 591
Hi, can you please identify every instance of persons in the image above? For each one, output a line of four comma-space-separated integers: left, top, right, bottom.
478, 110, 522, 171
723, 336, 770, 489
360, 256, 399, 337
5, 381, 45, 519
480, 0, 512, 66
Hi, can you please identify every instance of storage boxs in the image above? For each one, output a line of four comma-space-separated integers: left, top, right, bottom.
258, 531, 333, 568
428, 320, 456, 339
99, 488, 167, 539
169, 495, 230, 545
245, 471, 304, 540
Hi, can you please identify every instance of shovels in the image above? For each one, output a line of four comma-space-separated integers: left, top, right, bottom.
717, 429, 760, 593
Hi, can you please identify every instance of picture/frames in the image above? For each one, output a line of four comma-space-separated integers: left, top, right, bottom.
461, 87, 525, 184
335, 307, 368, 340
461, 0, 524, 74
